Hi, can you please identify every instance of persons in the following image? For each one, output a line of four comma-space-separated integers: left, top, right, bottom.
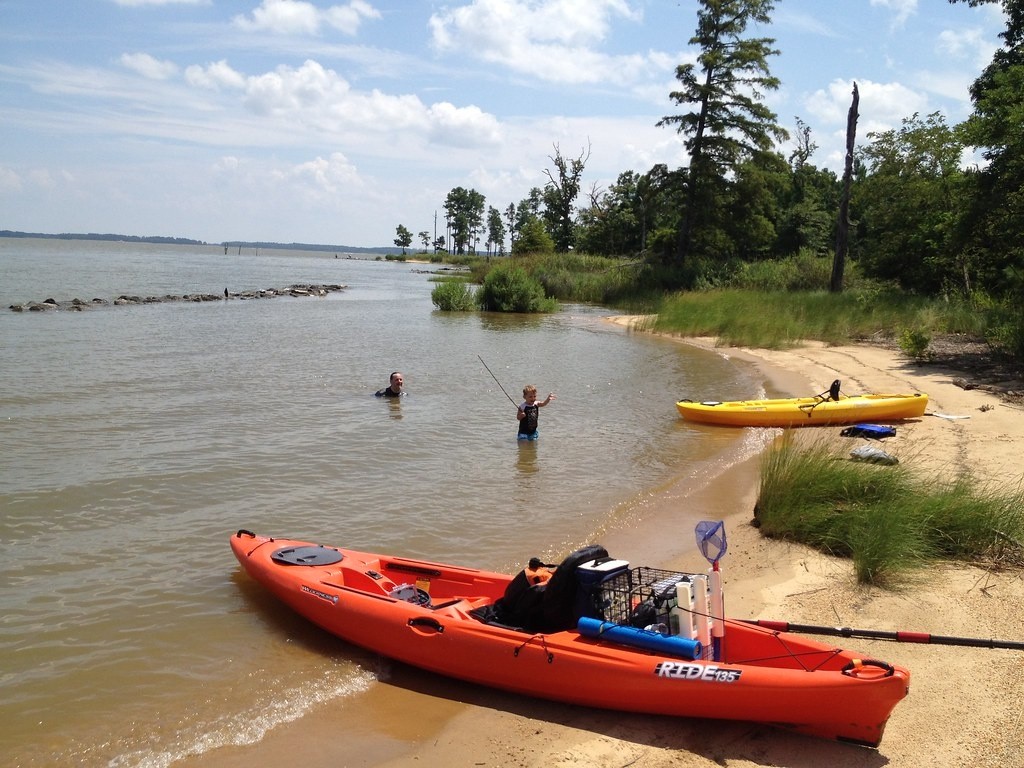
375, 372, 408, 398
516, 384, 557, 442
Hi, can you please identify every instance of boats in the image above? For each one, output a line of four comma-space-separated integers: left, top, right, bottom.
228, 529, 912, 748
675, 393, 930, 429
